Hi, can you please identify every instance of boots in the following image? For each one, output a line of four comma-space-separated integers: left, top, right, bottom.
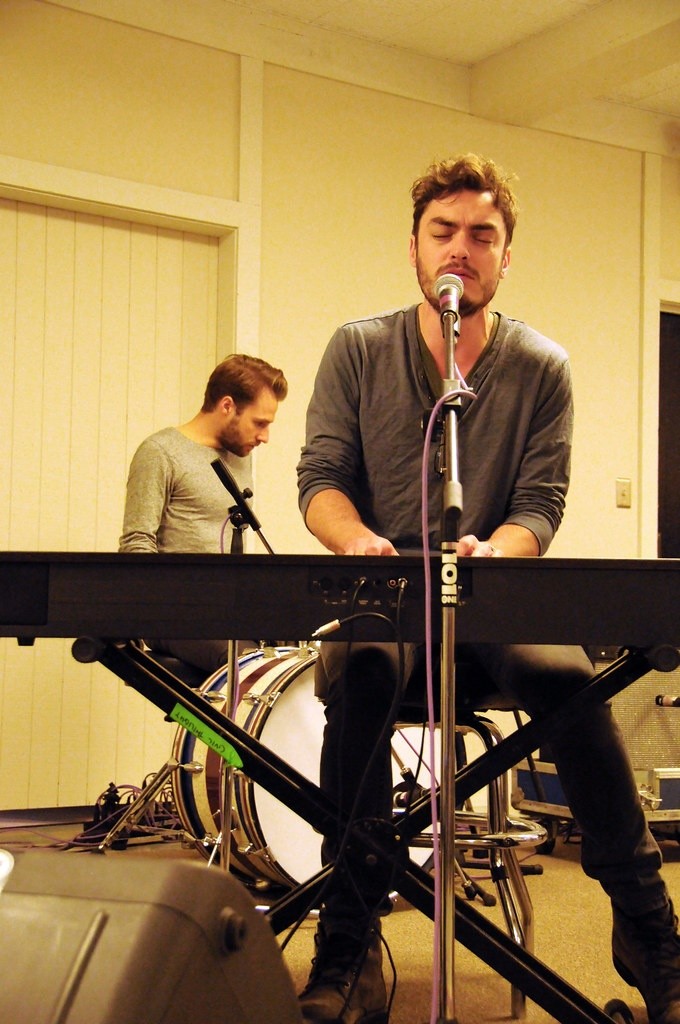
611, 899, 680, 1024
297, 919, 391, 1024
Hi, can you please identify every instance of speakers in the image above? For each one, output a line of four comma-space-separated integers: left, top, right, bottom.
0, 852, 304, 1024
579, 646, 680, 771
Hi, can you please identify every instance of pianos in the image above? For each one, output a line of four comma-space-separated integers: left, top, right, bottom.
0, 550, 680, 650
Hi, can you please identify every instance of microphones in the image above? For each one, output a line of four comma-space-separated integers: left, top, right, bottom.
434, 273, 464, 324
655, 694, 680, 708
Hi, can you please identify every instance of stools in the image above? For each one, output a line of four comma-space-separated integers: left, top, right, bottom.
323, 714, 547, 1019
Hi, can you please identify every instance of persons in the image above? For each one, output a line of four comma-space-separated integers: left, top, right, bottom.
297, 151, 680, 1024
118, 353, 292, 673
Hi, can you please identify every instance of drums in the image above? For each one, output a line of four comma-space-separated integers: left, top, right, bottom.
170, 641, 458, 907
